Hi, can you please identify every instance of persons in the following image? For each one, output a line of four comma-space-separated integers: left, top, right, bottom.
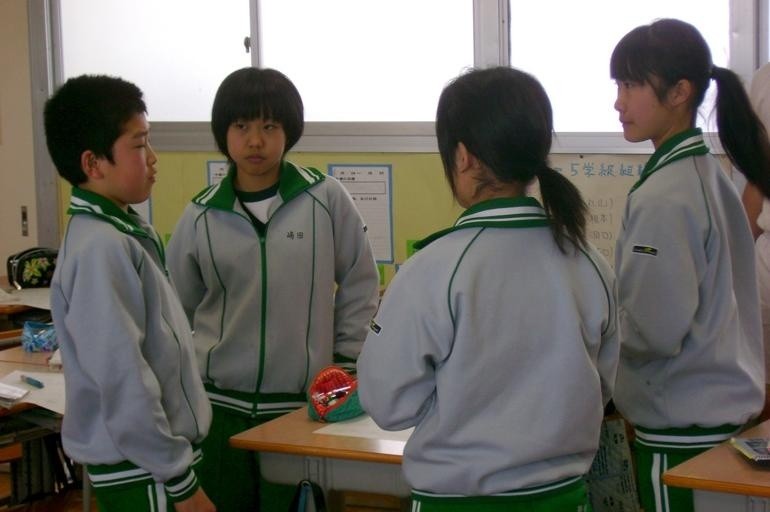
40, 75, 220, 512
351, 65, 624, 510
161, 65, 381, 512
735, 64, 769, 422
609, 17, 768, 512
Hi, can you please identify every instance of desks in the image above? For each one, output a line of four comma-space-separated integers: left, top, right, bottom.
228, 405, 415, 512
664, 417, 770, 512
0, 344, 91, 512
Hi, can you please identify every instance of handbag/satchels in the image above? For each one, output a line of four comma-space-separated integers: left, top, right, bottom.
6, 245, 55, 289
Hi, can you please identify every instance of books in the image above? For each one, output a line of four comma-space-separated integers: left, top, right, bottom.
0, 364, 67, 446
729, 436, 770, 467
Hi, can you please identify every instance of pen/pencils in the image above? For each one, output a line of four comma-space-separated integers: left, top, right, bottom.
21, 374, 43, 388
318, 386, 351, 401
